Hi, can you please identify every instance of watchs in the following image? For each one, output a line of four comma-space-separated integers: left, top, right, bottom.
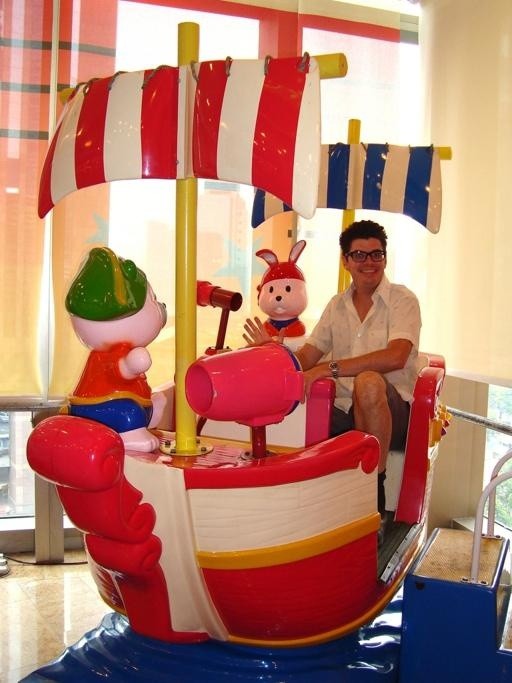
328, 359, 338, 379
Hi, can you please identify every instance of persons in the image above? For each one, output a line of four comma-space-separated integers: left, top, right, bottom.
240, 219, 427, 547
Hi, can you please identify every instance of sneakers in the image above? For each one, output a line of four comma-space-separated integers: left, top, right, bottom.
378, 486, 385, 520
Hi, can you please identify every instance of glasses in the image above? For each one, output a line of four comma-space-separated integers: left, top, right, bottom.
345, 250, 385, 262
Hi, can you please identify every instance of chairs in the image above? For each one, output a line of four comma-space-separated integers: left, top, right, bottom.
306, 351, 446, 525
